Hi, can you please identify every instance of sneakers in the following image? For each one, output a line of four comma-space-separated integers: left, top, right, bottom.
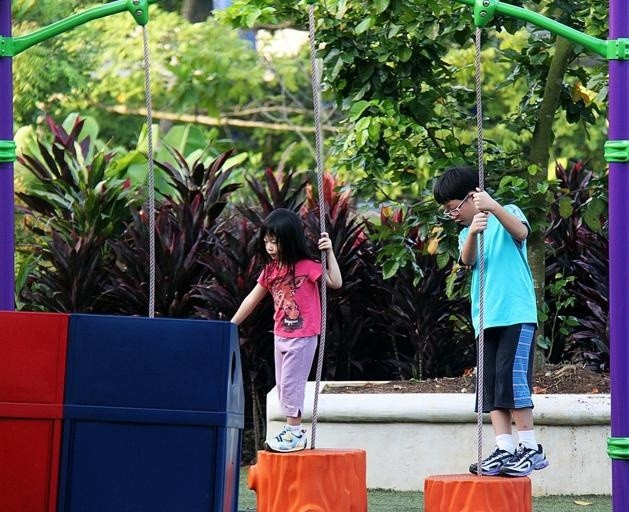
499, 442, 549, 477
469, 448, 517, 476
262, 426, 308, 454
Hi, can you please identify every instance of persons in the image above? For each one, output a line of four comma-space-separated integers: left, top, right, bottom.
225, 206, 344, 453
432, 164, 551, 478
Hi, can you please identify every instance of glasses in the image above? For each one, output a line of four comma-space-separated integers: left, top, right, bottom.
436, 195, 470, 220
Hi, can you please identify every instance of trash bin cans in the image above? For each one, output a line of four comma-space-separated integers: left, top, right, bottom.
58, 311, 243, 512
1, 312, 70, 512
